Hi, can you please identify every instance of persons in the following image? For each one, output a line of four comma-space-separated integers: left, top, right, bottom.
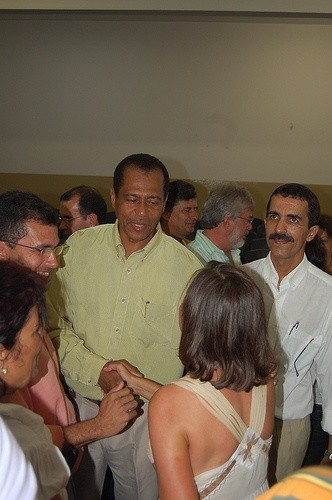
160, 181, 197, 246
41, 154, 205, 500
60, 185, 107, 237
240, 184, 332, 487
104, 260, 280, 500
0, 191, 138, 499
186, 187, 254, 265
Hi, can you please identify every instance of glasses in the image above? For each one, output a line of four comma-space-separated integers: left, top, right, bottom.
0, 239, 70, 258
57, 215, 84, 226
237, 216, 253, 225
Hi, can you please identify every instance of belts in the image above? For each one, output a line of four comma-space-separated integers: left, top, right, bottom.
84, 397, 102, 407
